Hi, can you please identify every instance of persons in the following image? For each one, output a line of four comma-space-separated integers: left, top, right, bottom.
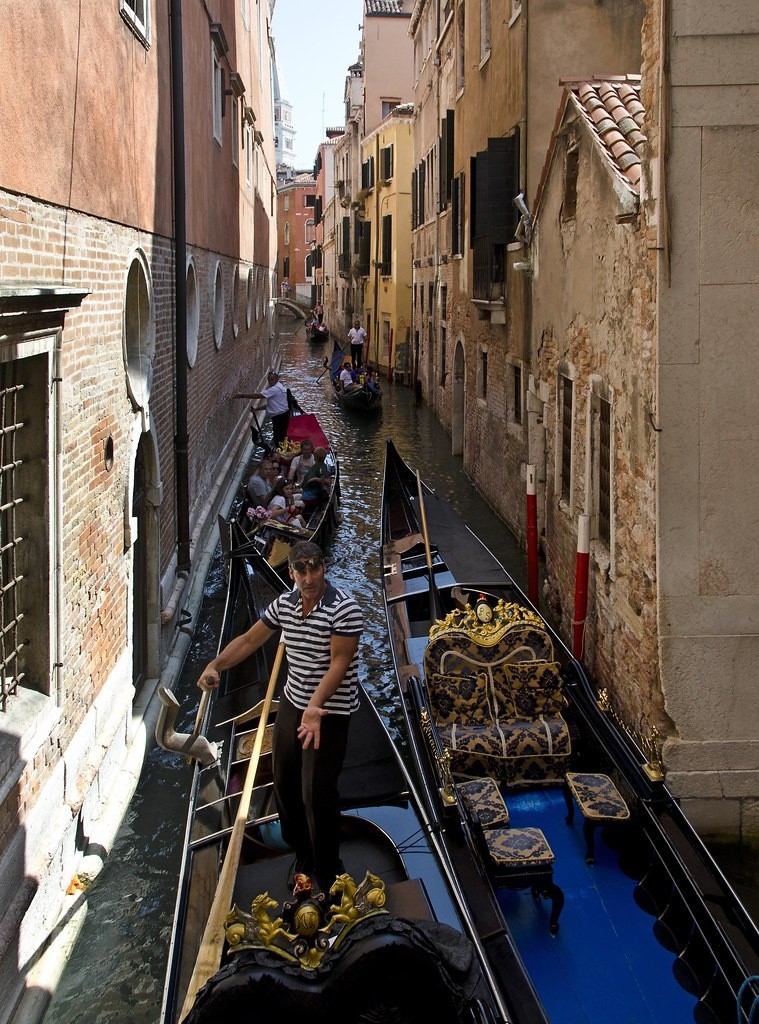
247, 439, 331, 529
338, 362, 375, 395
198, 541, 363, 895
309, 321, 326, 332
348, 321, 368, 370
233, 372, 289, 448
316, 302, 323, 323
281, 277, 292, 298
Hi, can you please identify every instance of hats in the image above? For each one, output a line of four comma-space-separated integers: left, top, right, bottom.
317, 300, 320, 303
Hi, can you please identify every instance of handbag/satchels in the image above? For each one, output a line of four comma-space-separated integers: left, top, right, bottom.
287, 515, 302, 528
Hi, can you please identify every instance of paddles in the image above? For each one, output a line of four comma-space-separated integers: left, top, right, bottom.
316, 336, 354, 382
415, 468, 444, 635
250, 405, 264, 442
295, 310, 314, 335
174, 627, 287, 1024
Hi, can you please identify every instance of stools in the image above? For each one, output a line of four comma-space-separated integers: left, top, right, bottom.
562, 769, 629, 866
483, 827, 565, 934
454, 779, 510, 829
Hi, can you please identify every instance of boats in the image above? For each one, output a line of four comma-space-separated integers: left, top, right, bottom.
324, 338, 383, 410
377, 435, 759, 1024
234, 389, 341, 577
306, 310, 330, 341
153, 518, 512, 1024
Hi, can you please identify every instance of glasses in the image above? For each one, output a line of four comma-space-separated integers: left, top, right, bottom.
347, 366, 351, 368
289, 557, 323, 571
268, 372, 278, 376
272, 466, 280, 470
285, 478, 288, 485
355, 324, 360, 325
313, 453, 315, 455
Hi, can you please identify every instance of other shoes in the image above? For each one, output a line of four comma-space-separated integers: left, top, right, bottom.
287, 857, 312, 891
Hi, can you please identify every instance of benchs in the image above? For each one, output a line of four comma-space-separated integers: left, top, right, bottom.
419, 592, 581, 787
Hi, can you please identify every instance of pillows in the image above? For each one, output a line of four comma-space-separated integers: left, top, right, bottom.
502, 661, 569, 721
430, 670, 495, 727
